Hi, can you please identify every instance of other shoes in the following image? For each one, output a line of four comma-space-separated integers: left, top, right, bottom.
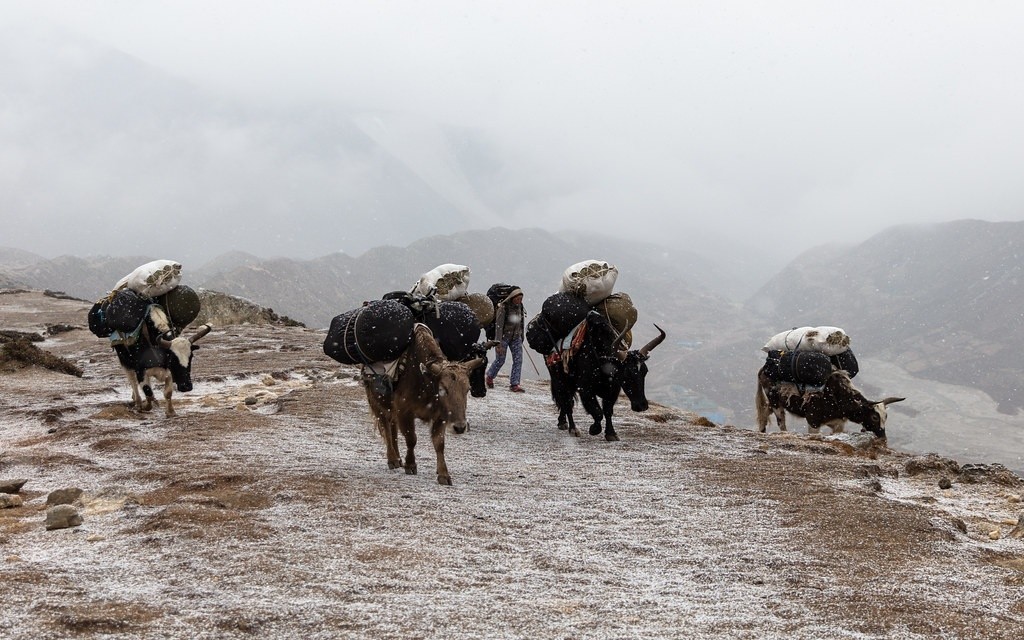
510, 384, 525, 393
487, 377, 494, 388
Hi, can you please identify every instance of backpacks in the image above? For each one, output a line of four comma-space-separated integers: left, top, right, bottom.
483, 283, 512, 342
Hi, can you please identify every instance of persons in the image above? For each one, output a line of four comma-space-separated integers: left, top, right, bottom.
485, 286, 527, 393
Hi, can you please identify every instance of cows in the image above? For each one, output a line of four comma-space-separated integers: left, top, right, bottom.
754, 358, 906, 440
110, 325, 212, 416
360, 291, 500, 476
545, 323, 667, 441
384, 292, 499, 398
